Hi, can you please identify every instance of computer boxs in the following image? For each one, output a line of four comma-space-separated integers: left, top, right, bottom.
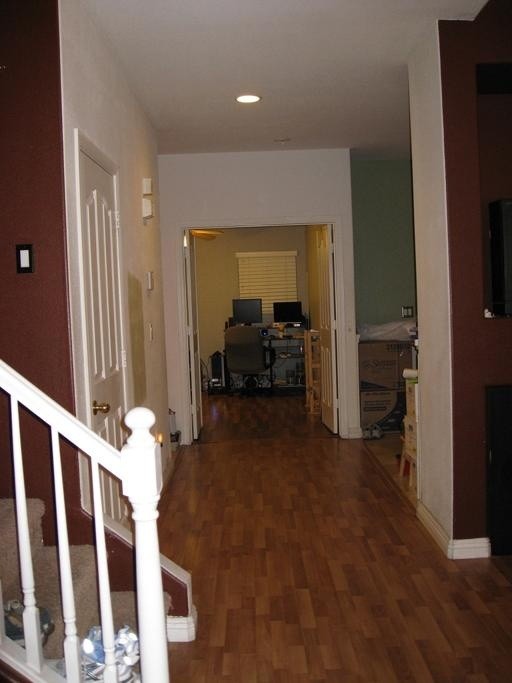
244, 374, 259, 391
212, 355, 230, 390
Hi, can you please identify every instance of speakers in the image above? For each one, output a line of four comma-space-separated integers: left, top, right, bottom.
229, 317, 236, 328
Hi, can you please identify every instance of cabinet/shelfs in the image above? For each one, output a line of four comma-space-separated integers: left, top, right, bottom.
305, 330, 321, 416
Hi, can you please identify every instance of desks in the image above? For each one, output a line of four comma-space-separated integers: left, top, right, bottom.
225, 332, 305, 397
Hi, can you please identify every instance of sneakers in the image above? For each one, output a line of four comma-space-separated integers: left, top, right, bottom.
363, 422, 372, 439
371, 423, 384, 437
3, 598, 140, 682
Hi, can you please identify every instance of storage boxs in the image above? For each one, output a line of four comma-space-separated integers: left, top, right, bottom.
358, 341, 419, 461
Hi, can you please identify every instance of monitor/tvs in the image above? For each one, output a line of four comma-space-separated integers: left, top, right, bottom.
233, 299, 262, 326
274, 302, 303, 327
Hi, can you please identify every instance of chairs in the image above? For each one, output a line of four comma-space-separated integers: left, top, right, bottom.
224, 325, 276, 399
